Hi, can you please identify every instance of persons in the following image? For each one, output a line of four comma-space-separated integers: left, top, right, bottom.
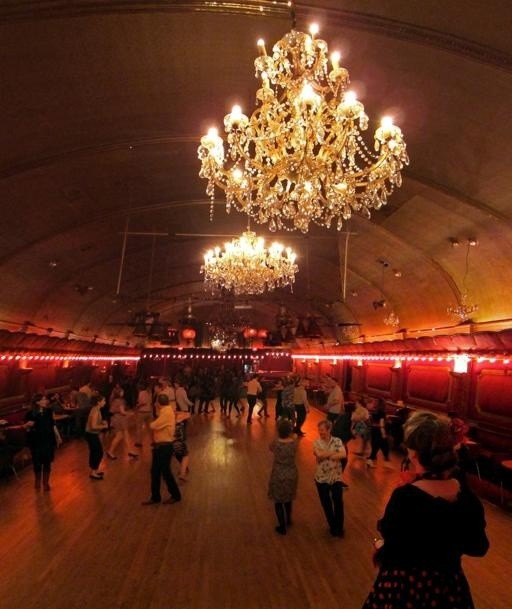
312, 421, 349, 535
22, 393, 62, 491
246, 374, 270, 424
272, 369, 309, 435
268, 421, 300, 534
72, 373, 143, 480
0, 420, 10, 431
177, 368, 246, 418
144, 378, 193, 505
360, 410, 490, 609
351, 398, 409, 468
325, 377, 345, 429
52, 392, 63, 413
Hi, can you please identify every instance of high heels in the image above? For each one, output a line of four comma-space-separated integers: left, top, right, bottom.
364, 459, 376, 469
128, 453, 139, 458
106, 453, 117, 461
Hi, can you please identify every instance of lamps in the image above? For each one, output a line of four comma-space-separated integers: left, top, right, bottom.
199, 213, 300, 294
391, 358, 402, 368
18, 359, 29, 368
182, 297, 197, 344
63, 360, 70, 368
301, 357, 339, 365
197, 1, 409, 233
357, 360, 363, 366
90, 360, 129, 368
242, 324, 271, 342
453, 352, 469, 373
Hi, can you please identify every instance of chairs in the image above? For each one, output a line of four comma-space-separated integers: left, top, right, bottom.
305, 380, 512, 489
0, 377, 132, 485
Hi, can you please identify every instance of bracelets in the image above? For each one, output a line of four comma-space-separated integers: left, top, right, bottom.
370, 537, 381, 550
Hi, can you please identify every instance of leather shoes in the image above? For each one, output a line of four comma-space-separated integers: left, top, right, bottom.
90, 472, 104, 480
141, 499, 160, 505
163, 497, 177, 504
276, 525, 286, 535
